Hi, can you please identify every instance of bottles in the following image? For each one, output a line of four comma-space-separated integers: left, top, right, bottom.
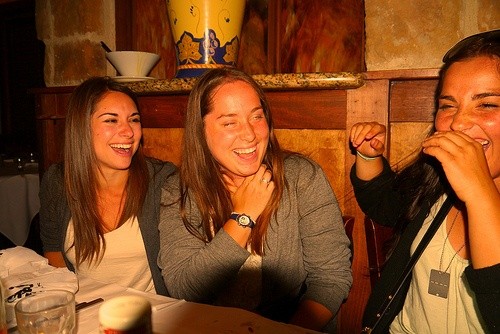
164, 0, 247, 79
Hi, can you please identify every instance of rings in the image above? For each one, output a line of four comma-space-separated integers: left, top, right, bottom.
261, 179, 270, 184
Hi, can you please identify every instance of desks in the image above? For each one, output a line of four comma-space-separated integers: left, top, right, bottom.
5, 276, 324, 334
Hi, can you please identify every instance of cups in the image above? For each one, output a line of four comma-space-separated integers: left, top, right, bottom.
14, 152, 38, 165
14, 290, 75, 334
98, 296, 152, 334
0, 279, 8, 334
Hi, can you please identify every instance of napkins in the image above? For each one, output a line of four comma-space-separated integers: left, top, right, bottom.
0, 264, 78, 322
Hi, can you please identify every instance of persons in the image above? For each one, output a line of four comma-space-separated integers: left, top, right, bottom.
349, 30, 500, 334
39, 75, 179, 298
156, 67, 352, 329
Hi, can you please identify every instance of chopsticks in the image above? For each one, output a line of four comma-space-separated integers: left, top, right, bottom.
7, 298, 104, 333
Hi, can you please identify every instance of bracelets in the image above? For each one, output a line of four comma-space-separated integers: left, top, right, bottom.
358, 151, 383, 161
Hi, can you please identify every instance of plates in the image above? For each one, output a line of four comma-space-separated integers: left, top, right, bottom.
110, 77, 156, 83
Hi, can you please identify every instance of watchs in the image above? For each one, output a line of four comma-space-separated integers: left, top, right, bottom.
229, 211, 256, 230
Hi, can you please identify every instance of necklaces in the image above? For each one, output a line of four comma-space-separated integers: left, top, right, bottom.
428, 207, 467, 299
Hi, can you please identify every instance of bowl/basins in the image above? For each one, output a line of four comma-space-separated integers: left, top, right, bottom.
105, 51, 161, 76
